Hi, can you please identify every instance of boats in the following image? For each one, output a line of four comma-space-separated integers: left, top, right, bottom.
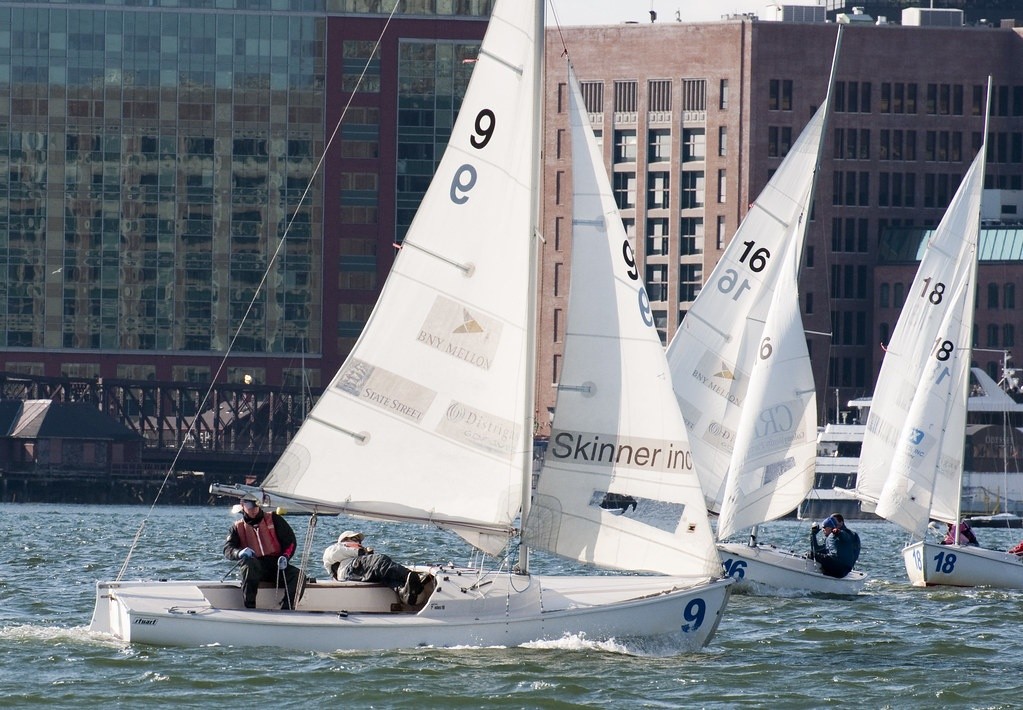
793, 362, 1023, 522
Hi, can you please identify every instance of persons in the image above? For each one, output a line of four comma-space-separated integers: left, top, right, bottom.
803, 513, 860, 578
945, 521, 979, 547
223, 494, 306, 610
323, 531, 431, 605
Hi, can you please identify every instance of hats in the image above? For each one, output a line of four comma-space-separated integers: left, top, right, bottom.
822, 518, 837, 529
338, 530, 364, 543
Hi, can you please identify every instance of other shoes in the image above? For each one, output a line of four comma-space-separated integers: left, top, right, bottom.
417, 574, 433, 595
403, 572, 418, 605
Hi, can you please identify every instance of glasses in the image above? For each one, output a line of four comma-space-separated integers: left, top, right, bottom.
349, 536, 362, 545
243, 501, 260, 507
822, 526, 828, 529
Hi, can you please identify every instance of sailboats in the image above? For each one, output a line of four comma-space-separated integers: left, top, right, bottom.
87, 0, 749, 662
847, 74, 1023, 592
665, 20, 870, 600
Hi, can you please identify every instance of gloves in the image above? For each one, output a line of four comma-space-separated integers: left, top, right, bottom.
237, 547, 255, 557
941, 541, 946, 544
278, 556, 287, 570
811, 524, 822, 535
811, 538, 818, 550
